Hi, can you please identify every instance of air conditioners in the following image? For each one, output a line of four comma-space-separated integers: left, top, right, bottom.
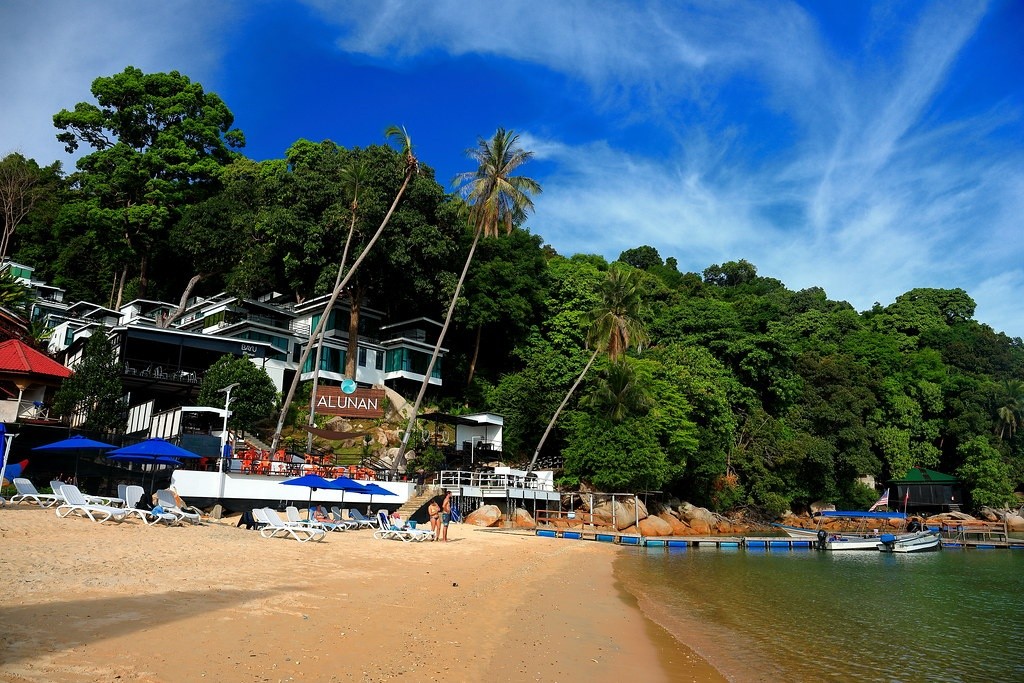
218, 321, 224, 328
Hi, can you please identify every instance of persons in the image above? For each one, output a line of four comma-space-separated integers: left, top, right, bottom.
428, 499, 441, 541
223, 441, 233, 471
442, 491, 452, 541
314, 504, 336, 523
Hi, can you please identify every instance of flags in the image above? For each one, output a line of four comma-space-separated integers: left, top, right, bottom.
869, 488, 889, 510
904, 488, 910, 505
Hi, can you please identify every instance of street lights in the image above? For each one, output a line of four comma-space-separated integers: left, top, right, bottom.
218, 397, 237, 498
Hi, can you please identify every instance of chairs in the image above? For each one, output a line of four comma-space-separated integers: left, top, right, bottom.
8, 476, 436, 543
178, 450, 376, 481
124, 361, 204, 386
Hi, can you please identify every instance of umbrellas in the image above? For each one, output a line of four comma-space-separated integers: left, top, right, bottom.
279, 474, 344, 522
0, 422, 5, 468
31, 434, 120, 485
345, 483, 399, 520
105, 437, 201, 505
107, 454, 182, 494
329, 476, 372, 521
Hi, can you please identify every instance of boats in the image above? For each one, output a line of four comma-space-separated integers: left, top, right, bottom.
770, 510, 907, 551
876, 526, 940, 553
